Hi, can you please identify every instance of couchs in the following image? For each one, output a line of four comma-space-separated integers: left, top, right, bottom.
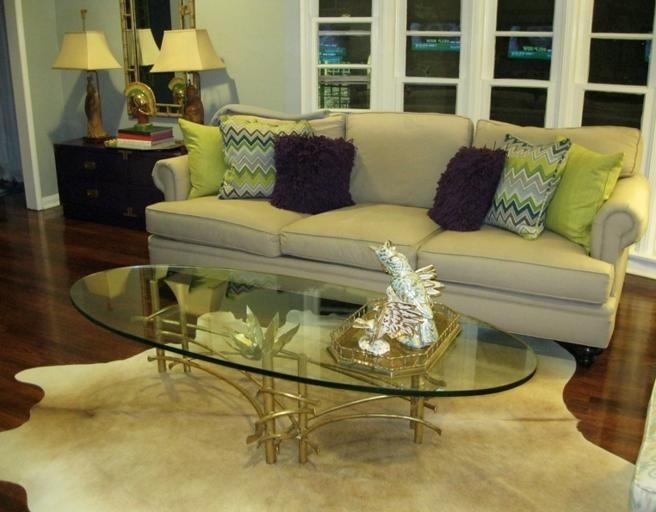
145, 105, 650, 368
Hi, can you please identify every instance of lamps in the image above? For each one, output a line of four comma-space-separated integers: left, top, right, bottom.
149, 6, 224, 125
125, 28, 160, 78
52, 10, 122, 143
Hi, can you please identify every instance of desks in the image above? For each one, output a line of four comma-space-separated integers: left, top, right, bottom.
53, 137, 187, 229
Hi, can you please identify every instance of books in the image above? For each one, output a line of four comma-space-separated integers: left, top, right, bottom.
116, 124, 175, 150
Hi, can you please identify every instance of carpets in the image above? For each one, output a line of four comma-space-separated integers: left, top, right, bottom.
0, 315, 636, 512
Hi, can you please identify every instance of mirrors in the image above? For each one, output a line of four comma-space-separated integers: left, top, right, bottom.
119, 0, 201, 118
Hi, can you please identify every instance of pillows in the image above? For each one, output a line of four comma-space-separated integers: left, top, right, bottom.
427, 147, 506, 231
217, 114, 311, 198
271, 135, 353, 212
178, 118, 225, 198
546, 136, 623, 250
483, 133, 570, 238
